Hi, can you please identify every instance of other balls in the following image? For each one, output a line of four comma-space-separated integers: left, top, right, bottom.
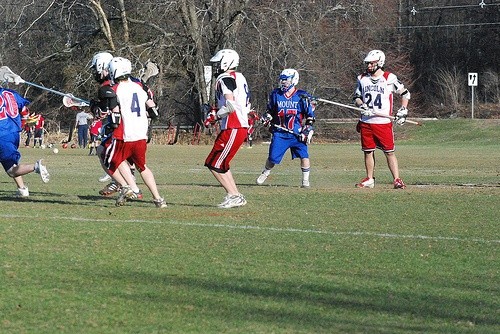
53, 148, 58, 154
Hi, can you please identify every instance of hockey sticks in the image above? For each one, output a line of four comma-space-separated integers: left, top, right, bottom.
0, 66, 89, 106
63, 93, 91, 107
315, 96, 422, 128
250, 112, 304, 138
139, 63, 160, 83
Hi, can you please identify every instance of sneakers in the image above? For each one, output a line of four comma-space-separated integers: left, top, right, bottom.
217, 192, 247, 208
153, 196, 168, 209
302, 180, 310, 187
355, 176, 376, 188
256, 171, 270, 185
9, 186, 30, 197
115, 185, 133, 207
394, 178, 406, 188
99, 182, 120, 196
33, 159, 50, 183
98, 173, 113, 182
129, 189, 144, 200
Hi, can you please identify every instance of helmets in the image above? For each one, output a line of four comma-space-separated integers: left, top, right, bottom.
107, 56, 132, 78
91, 53, 113, 74
279, 69, 300, 85
210, 48, 239, 71
364, 49, 386, 67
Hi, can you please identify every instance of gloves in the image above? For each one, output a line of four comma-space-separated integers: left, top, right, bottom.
91, 121, 112, 137
204, 112, 219, 128
259, 113, 273, 127
394, 106, 408, 126
357, 104, 375, 117
89, 98, 108, 119
298, 125, 314, 145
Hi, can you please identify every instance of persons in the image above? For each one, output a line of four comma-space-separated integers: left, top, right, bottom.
354, 50, 411, 190
33, 112, 45, 147
101, 56, 167, 207
25, 112, 35, 148
257, 69, 316, 187
202, 50, 251, 208
247, 110, 259, 147
75, 109, 101, 155
90, 52, 136, 195
0, 87, 49, 196
